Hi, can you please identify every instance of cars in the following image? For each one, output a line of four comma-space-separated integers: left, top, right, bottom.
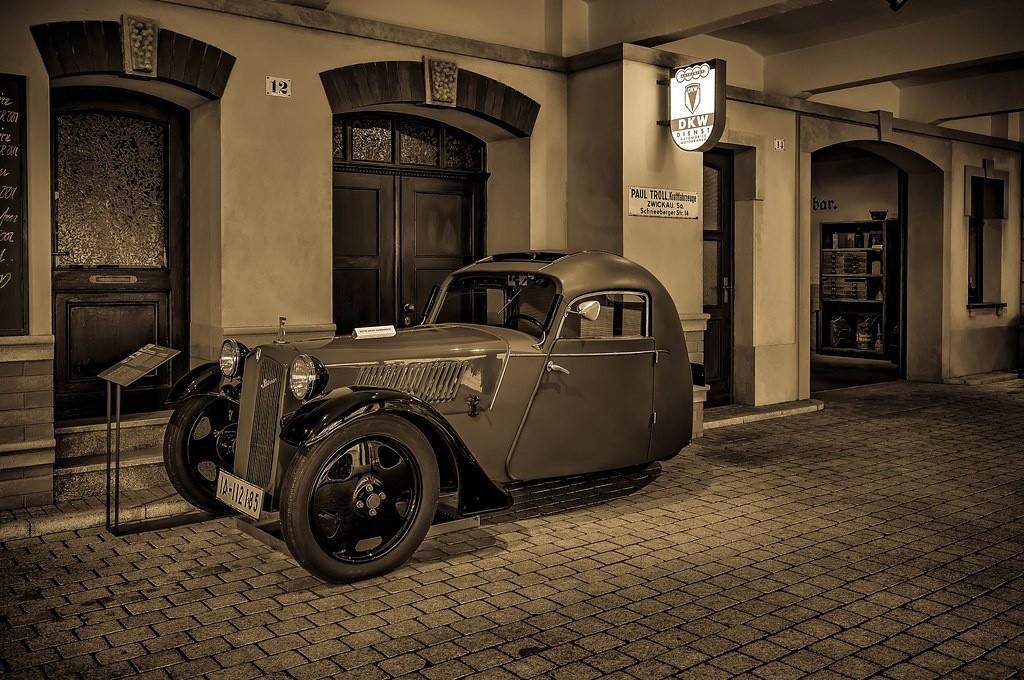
164, 247, 696, 585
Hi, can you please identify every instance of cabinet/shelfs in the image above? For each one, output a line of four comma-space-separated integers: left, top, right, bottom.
817, 220, 897, 358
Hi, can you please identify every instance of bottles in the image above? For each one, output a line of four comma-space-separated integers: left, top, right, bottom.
875, 333, 883, 351
